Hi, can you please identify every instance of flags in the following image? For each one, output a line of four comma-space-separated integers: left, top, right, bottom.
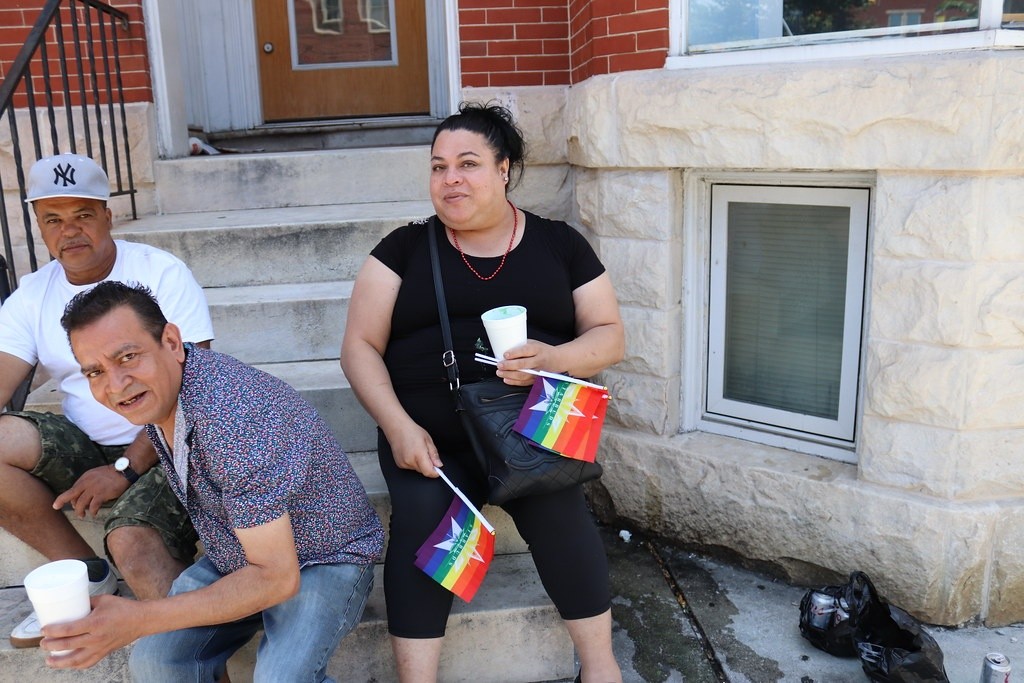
512, 376, 609, 464
413, 493, 495, 604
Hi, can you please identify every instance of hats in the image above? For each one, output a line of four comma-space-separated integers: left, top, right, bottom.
24, 154, 111, 201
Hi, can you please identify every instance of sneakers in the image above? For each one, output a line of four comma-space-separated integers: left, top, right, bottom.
9, 558, 121, 648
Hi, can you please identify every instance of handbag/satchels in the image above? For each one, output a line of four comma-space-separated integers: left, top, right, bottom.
799, 571, 950, 683
454, 369, 603, 504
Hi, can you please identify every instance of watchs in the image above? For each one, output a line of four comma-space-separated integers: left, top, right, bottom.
115, 456, 140, 484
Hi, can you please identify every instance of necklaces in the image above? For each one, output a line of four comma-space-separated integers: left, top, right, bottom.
449, 200, 519, 280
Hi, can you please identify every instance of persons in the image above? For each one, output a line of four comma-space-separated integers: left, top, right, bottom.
0, 152, 215, 649
339, 99, 626, 683
39, 280, 388, 683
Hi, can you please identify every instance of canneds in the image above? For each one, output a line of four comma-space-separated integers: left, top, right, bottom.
979, 652, 1011, 682
806, 592, 849, 628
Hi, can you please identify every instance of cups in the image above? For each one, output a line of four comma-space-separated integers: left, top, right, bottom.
481, 305, 528, 359
23, 559, 92, 658
